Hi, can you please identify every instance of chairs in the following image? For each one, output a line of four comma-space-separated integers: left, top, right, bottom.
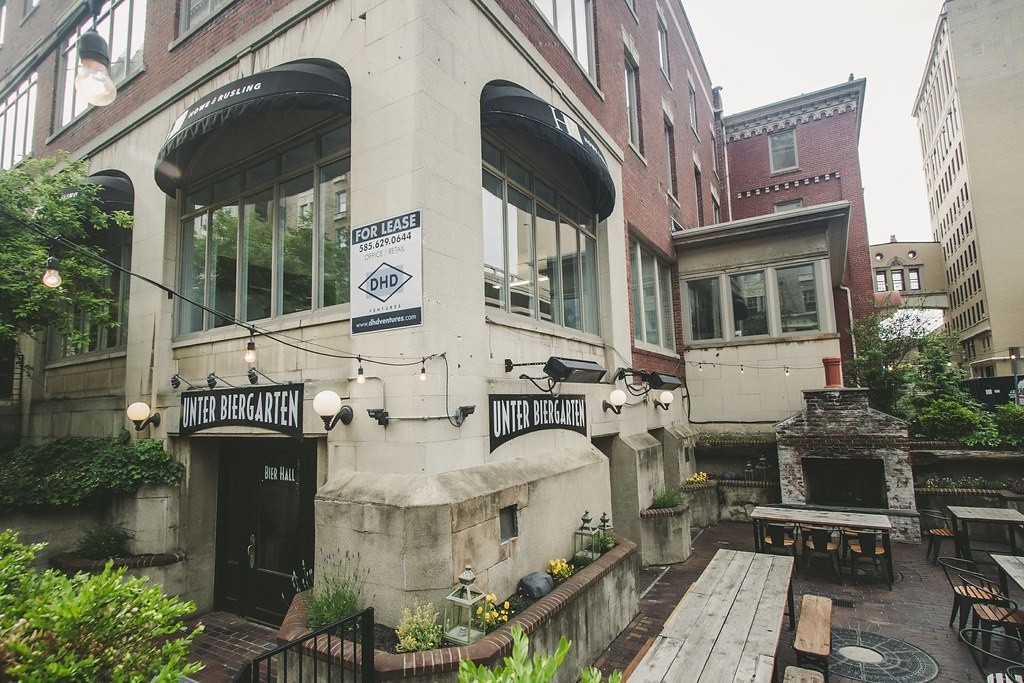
937, 555, 1024, 683
916, 508, 969, 567
761, 523, 894, 592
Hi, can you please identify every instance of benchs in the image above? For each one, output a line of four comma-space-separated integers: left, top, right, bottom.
620, 579, 833, 683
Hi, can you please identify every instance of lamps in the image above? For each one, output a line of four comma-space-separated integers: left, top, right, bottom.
612, 367, 683, 391
247, 367, 284, 386
171, 372, 197, 389
367, 408, 390, 425
206, 373, 235, 389
314, 390, 353, 431
573, 509, 614, 566
654, 392, 675, 412
442, 565, 487, 647
457, 405, 475, 424
127, 402, 161, 431
603, 389, 627, 414
505, 356, 607, 393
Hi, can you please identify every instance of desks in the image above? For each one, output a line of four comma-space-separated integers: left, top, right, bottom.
750, 506, 894, 584
946, 505, 1024, 564
999, 490, 1024, 556
989, 553, 1024, 635
625, 549, 795, 683
